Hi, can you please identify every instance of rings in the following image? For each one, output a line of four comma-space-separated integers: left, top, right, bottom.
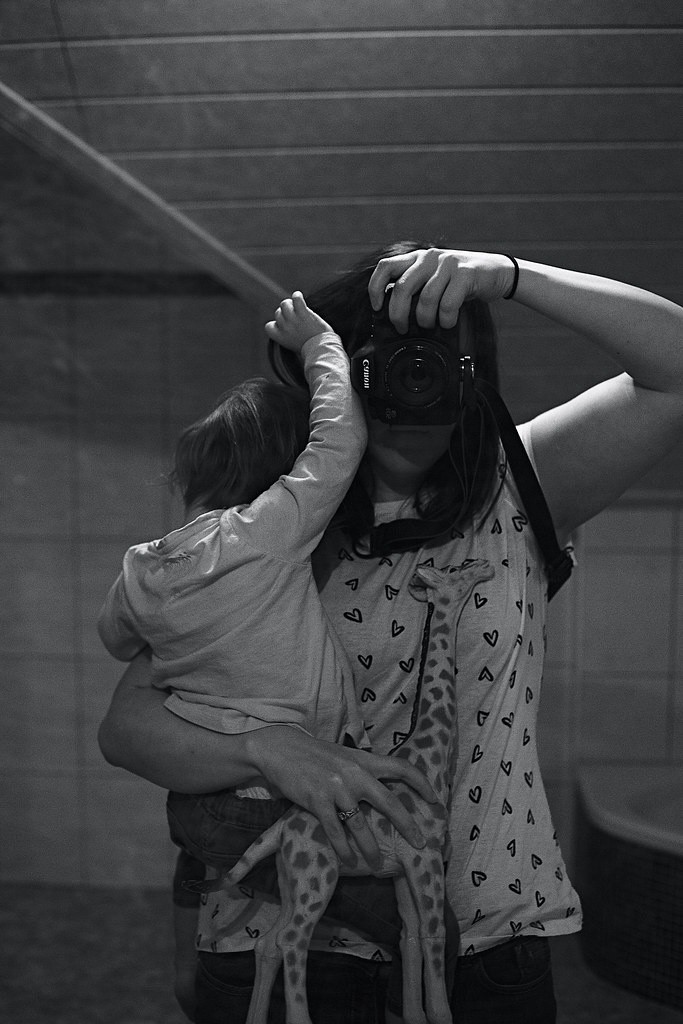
338, 806, 362, 821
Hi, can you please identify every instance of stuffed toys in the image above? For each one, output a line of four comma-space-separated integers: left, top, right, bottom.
179, 557, 496, 1024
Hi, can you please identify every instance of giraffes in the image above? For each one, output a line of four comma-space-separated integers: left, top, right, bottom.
218, 557, 495, 1024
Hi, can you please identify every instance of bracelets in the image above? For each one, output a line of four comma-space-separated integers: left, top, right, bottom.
503, 254, 520, 300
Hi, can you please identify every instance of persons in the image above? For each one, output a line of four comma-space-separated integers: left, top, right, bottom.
99, 241, 683, 1024
101, 291, 460, 1024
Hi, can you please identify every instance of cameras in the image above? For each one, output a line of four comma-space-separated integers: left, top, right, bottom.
350, 283, 475, 427
